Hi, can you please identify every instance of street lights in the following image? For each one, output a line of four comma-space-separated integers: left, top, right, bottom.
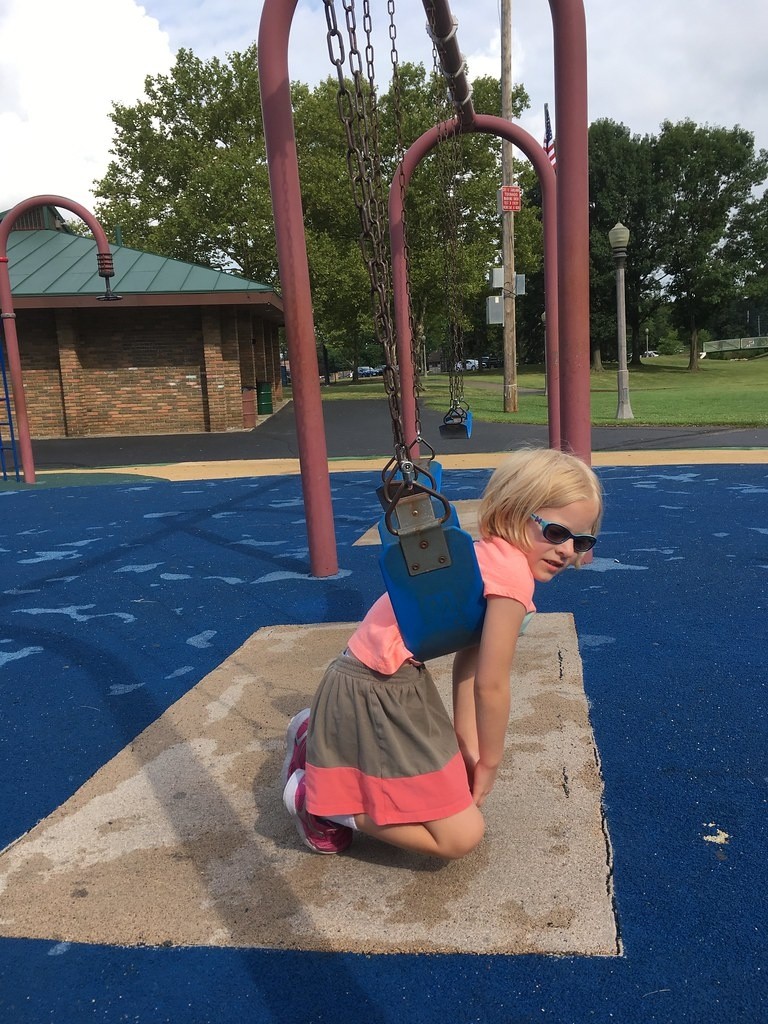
608, 224, 635, 418
645, 328, 649, 358
541, 311, 548, 396
422, 335, 427, 377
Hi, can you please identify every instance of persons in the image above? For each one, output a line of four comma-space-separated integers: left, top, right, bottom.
281, 440, 606, 860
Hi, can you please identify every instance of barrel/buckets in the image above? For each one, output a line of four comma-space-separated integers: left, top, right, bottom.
256, 382, 273, 415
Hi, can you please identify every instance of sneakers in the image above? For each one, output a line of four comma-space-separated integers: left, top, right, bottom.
281, 707, 311, 787
283, 768, 353, 854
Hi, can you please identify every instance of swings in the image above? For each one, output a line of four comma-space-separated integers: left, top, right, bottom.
319, 0, 491, 664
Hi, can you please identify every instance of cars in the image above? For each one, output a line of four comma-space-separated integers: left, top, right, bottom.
338, 364, 422, 378
455, 355, 504, 373
628, 350, 659, 360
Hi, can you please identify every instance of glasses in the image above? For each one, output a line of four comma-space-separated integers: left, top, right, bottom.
529, 511, 597, 553
